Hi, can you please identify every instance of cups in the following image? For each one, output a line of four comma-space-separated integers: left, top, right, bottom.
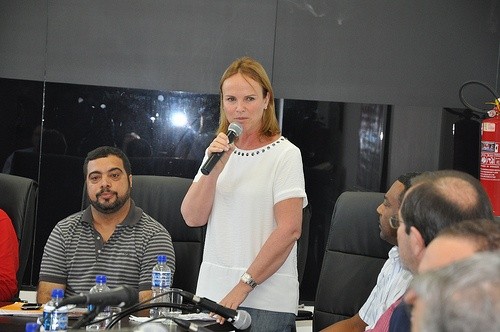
171, 288, 183, 314
105, 307, 122, 329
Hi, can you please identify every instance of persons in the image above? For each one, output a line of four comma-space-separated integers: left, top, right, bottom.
0, 209, 20, 301
36, 145, 175, 316
181, 55, 308, 332
316, 169, 500, 332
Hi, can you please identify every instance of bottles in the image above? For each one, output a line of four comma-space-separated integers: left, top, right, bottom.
150, 256, 172, 316
25, 322, 40, 332
86, 274, 111, 332
43, 290, 69, 332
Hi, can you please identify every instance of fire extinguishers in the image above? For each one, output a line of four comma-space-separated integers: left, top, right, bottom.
459, 81, 500, 216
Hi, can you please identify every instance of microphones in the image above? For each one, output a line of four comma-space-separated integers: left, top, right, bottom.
60, 283, 139, 309
178, 290, 253, 330
200, 121, 243, 175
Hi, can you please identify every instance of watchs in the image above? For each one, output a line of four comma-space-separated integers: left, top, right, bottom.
241, 272, 257, 288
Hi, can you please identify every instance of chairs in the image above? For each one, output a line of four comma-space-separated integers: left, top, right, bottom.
313, 190, 393, 332
83, 175, 207, 305
0, 173, 38, 302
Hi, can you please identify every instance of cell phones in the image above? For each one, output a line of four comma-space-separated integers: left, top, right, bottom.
21, 303, 40, 310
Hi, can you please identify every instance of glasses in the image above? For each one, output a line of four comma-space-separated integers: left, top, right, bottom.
389, 216, 406, 229
